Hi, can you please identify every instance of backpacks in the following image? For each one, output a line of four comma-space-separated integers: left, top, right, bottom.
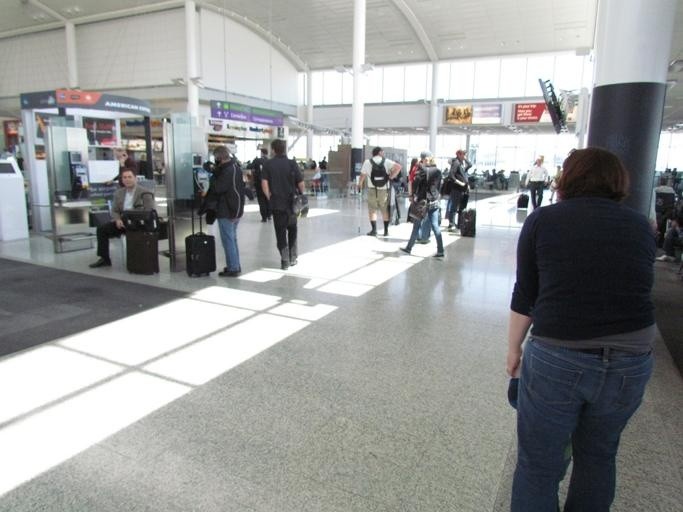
368, 157, 389, 188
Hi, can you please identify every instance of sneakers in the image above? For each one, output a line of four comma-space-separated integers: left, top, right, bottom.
223, 266, 241, 273
433, 254, 444, 259
218, 271, 238, 277
290, 259, 298, 266
399, 247, 411, 255
281, 260, 289, 271
367, 232, 377, 236
655, 254, 677, 263
384, 233, 389, 236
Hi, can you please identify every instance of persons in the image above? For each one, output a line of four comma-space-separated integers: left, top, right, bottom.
548, 165, 561, 201
292, 155, 327, 170
504, 146, 656, 512
246, 148, 271, 222
356, 145, 506, 260
653, 167, 683, 261
261, 138, 305, 271
202, 145, 245, 277
88, 168, 156, 268
105, 148, 137, 187
524, 157, 547, 209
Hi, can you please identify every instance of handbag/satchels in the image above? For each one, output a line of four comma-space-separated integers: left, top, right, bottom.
406, 199, 428, 224
292, 195, 305, 215
441, 177, 451, 196
122, 209, 160, 232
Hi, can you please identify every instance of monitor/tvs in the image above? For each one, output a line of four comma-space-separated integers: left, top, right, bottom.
87, 160, 120, 184
537, 78, 570, 135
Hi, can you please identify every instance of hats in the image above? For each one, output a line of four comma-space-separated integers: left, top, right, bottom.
456, 150, 466, 155
421, 150, 433, 159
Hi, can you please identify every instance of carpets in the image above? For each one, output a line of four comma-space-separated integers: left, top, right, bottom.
649, 245, 683, 378
466, 192, 499, 203
0, 255, 194, 359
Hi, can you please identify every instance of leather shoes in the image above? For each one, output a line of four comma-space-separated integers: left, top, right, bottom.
89, 258, 112, 268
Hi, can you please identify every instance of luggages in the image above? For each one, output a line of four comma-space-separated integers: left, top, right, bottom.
460, 205, 476, 237
516, 194, 529, 209
184, 231, 217, 278
126, 229, 160, 276
